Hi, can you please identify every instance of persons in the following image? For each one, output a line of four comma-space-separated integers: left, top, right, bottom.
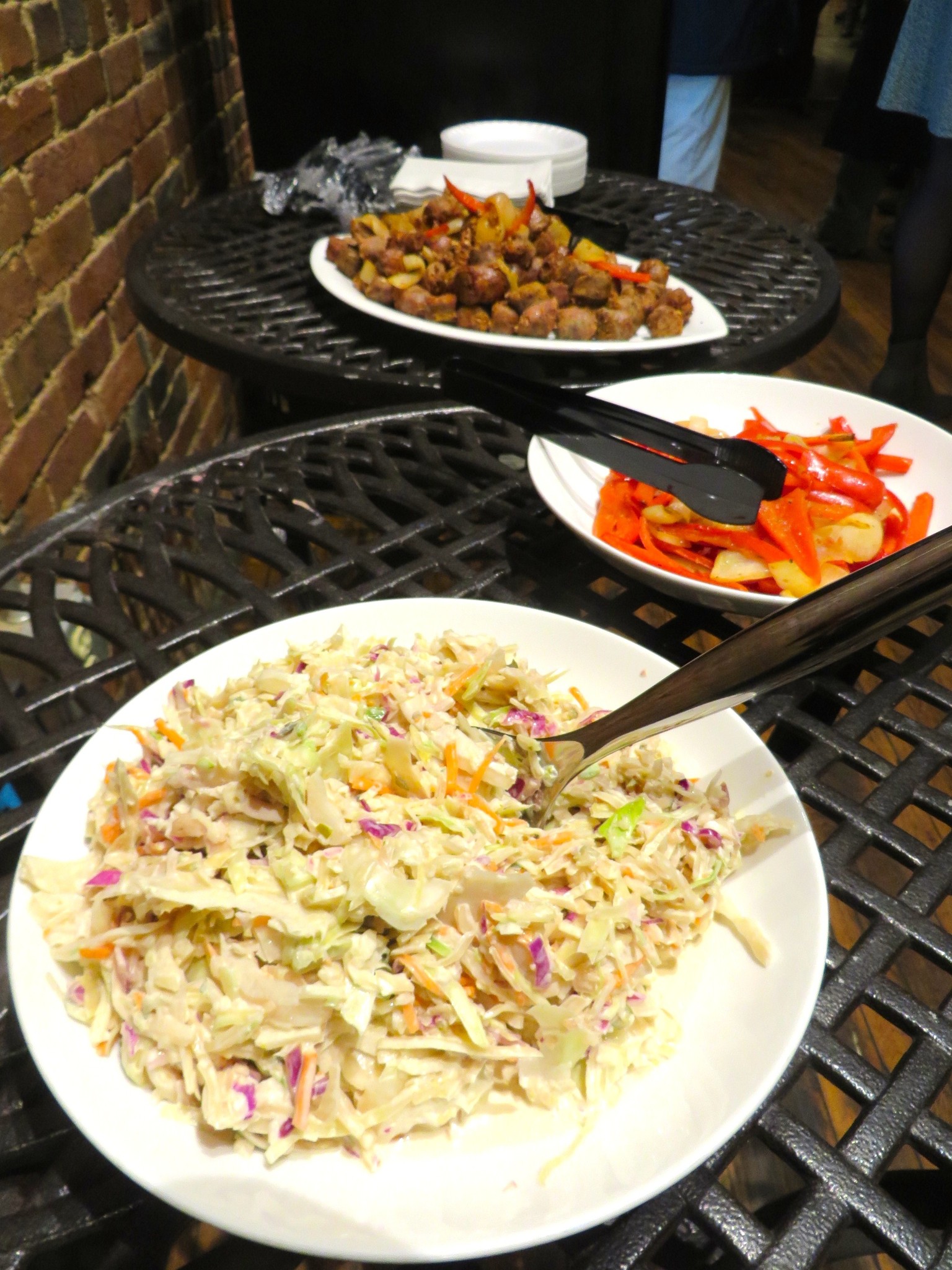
863, 0, 952, 418
809, 0, 931, 265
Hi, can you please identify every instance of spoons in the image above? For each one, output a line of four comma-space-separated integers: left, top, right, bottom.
474, 522, 951, 824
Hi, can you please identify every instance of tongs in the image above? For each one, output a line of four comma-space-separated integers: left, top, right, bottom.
436, 356, 786, 526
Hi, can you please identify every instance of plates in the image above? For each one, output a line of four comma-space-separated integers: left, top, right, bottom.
525, 370, 952, 611
17, 593, 833, 1266
309, 234, 729, 353
435, 119, 591, 198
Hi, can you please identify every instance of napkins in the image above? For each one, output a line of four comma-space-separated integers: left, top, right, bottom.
389, 156, 555, 214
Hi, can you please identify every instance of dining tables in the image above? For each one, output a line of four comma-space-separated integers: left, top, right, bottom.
126, 146, 844, 595
0, 392, 952, 1268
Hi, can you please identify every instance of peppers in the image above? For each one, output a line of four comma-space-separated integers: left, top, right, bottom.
595, 410, 935, 599
